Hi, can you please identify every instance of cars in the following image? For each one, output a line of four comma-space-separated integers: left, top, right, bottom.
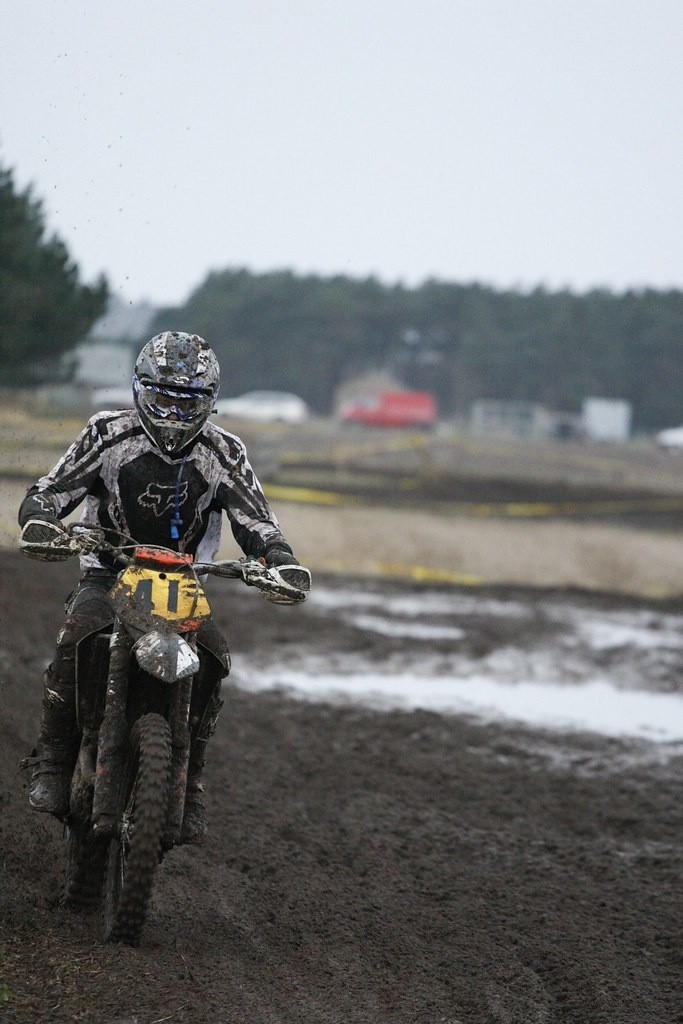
339, 379, 438, 435
476, 395, 683, 455
212, 386, 311, 429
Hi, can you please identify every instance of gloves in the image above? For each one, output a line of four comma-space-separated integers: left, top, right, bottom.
264, 549, 299, 566
29, 514, 62, 529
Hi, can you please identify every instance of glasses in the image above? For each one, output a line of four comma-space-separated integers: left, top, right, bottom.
138, 385, 210, 420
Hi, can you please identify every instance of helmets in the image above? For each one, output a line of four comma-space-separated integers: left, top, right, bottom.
132, 330, 221, 455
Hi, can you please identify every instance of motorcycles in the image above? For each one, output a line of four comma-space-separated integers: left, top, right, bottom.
17, 514, 312, 947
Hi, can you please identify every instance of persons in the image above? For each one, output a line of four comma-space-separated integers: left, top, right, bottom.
18, 330, 300, 844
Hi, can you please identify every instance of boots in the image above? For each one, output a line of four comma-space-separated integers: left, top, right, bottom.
181, 714, 221, 839
28, 664, 75, 814
